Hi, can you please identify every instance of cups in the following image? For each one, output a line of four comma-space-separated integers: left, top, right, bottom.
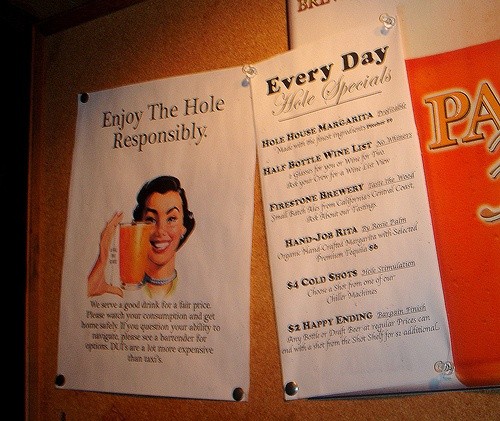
118, 222, 151, 291
